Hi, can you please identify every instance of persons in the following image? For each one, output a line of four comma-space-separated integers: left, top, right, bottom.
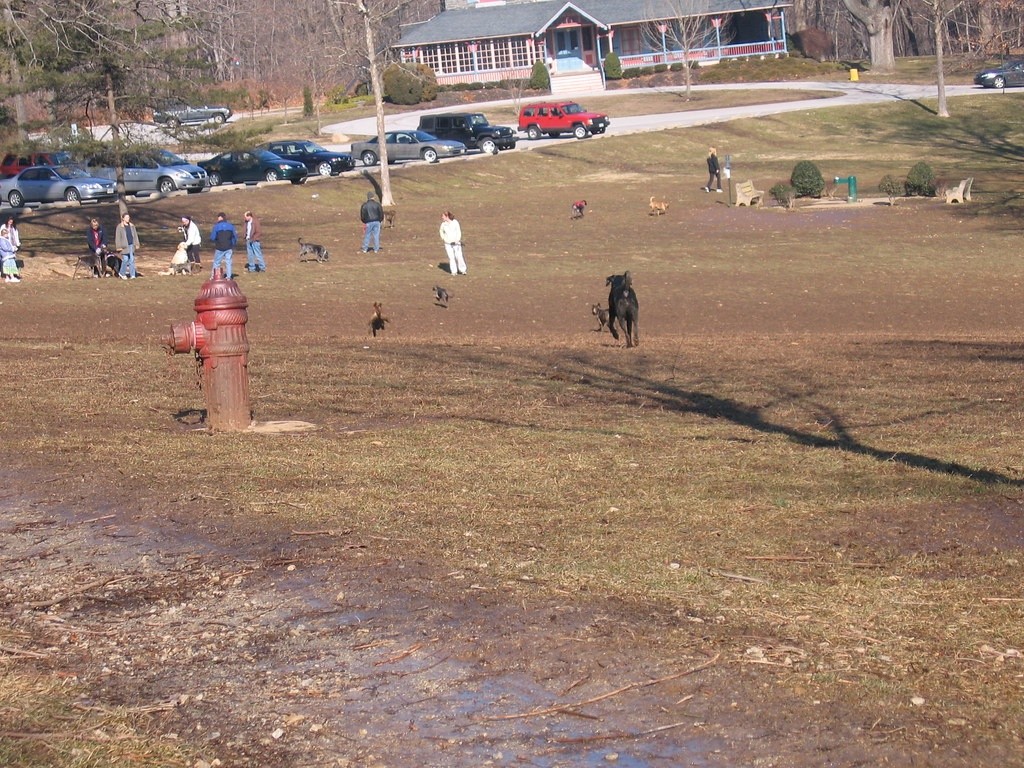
106, 249, 122, 277
243, 210, 267, 272
0, 216, 21, 282
115, 213, 140, 280
210, 212, 237, 279
178, 216, 201, 264
360, 192, 383, 253
704, 147, 724, 193
439, 211, 466, 275
87, 219, 110, 277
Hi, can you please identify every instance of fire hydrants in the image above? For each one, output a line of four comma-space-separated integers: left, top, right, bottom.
159, 267, 254, 432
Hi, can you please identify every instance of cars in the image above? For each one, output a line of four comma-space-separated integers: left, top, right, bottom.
973, 58, 1024, 89
152, 104, 233, 129
196, 146, 310, 187
245, 138, 357, 176
0, 164, 118, 208
351, 129, 468, 166
87, 147, 209, 195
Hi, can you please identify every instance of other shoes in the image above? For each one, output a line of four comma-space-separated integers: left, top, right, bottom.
93, 275, 98, 278
118, 272, 128, 280
717, 189, 722, 193
5, 277, 20, 283
704, 187, 709, 193
105, 273, 110, 277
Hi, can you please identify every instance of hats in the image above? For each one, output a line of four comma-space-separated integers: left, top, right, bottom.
366, 191, 374, 199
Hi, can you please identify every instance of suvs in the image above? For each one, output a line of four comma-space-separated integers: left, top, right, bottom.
0, 152, 87, 180
517, 101, 611, 139
416, 111, 518, 153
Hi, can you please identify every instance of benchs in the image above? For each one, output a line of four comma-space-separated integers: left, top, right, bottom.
944, 177, 975, 204
734, 180, 764, 207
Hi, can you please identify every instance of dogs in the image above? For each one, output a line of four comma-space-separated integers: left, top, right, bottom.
590, 270, 640, 349
648, 196, 673, 217
368, 302, 391, 338
570, 199, 587, 220
158, 242, 201, 275
381, 210, 396, 229
432, 284, 456, 308
298, 238, 329, 264
72, 251, 116, 280
